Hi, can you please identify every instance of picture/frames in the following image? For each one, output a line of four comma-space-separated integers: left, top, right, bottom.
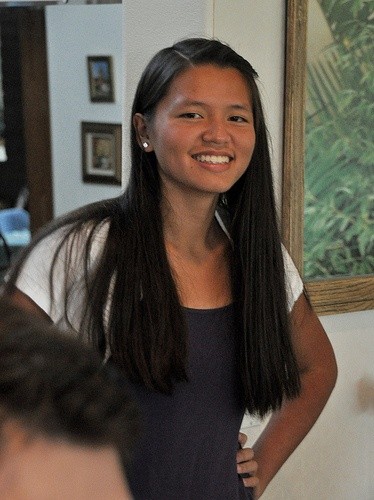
279, 0, 374, 315
79, 120, 123, 185
86, 54, 115, 103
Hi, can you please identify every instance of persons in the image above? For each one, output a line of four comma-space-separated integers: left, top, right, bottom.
0, 38, 338, 499
